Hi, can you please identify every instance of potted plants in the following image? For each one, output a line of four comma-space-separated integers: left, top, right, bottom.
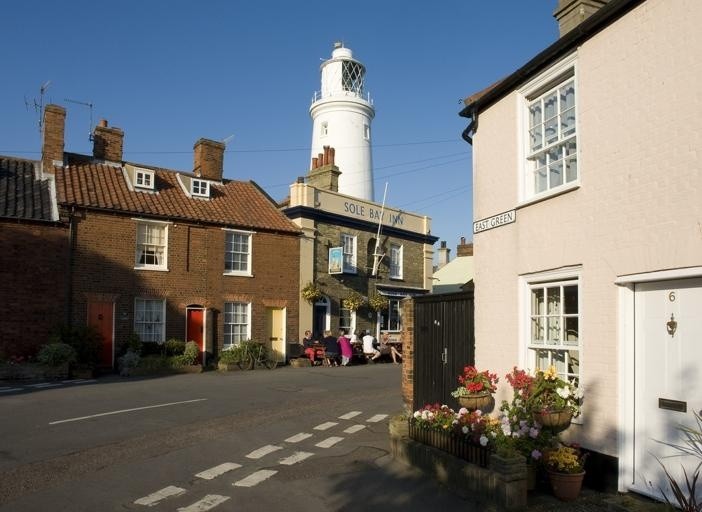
29, 322, 107, 382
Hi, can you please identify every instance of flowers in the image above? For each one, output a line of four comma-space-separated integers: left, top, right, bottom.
450, 364, 599, 472
406, 401, 501, 450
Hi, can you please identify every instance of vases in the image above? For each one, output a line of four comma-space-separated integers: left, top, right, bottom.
549, 469, 585, 501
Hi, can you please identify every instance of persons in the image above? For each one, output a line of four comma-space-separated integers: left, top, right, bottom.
379, 331, 402, 365
319, 330, 340, 368
336, 331, 353, 367
361, 328, 382, 364
303, 330, 323, 367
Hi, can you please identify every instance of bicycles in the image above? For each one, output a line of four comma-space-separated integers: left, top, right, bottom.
236, 335, 280, 372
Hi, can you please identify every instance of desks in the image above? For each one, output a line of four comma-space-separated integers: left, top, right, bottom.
308, 340, 403, 366
